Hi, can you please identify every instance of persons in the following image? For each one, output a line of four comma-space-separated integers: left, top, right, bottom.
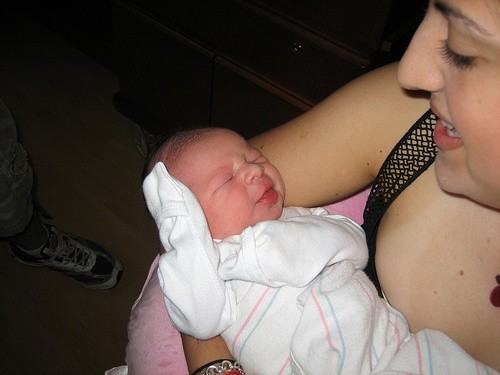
0, 94, 124, 292
143, 125, 500, 375
176, 1, 500, 375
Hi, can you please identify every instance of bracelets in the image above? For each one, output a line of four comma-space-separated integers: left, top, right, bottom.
191, 359, 245, 375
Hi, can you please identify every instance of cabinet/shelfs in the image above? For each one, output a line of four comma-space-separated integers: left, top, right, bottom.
112, 0, 366, 148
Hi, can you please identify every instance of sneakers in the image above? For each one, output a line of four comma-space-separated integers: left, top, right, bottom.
12, 220, 124, 292
